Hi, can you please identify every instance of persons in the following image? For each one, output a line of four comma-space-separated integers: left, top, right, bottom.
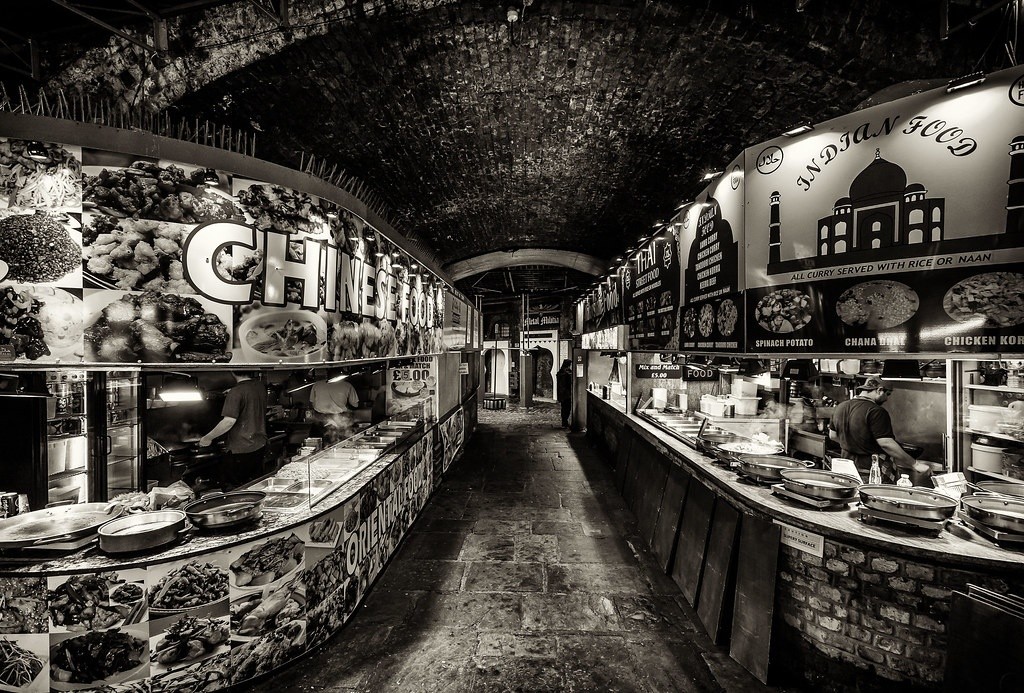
309, 368, 359, 427
556, 359, 572, 429
829, 376, 930, 484
197, 371, 268, 492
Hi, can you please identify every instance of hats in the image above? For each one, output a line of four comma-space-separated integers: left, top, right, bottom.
856, 376, 894, 391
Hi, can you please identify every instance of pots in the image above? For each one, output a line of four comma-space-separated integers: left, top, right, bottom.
698, 433, 1024, 536
183, 489, 267, 530
0, 500, 123, 550
96, 509, 193, 554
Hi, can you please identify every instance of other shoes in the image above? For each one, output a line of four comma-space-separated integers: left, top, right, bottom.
561, 424, 570, 428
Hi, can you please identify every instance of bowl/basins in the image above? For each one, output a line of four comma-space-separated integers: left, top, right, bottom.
238, 310, 328, 362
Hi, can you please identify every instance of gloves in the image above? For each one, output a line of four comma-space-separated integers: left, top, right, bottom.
913, 460, 933, 476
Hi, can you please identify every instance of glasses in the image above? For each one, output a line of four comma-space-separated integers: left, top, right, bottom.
882, 390, 891, 397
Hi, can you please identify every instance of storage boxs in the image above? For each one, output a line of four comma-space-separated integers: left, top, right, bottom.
727, 374, 762, 414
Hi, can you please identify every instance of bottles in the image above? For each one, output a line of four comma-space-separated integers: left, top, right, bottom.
868, 454, 881, 485
897, 473, 913, 487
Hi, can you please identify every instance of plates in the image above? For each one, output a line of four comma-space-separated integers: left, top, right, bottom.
0, 512, 362, 693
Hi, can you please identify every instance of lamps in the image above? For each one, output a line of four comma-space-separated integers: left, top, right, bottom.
781, 122, 813, 137
158, 364, 385, 402
327, 203, 338, 217
366, 226, 448, 291
203, 168, 218, 185
574, 220, 669, 304
27, 141, 48, 159
947, 70, 985, 92
599, 352, 921, 383
673, 197, 695, 211
702, 168, 724, 182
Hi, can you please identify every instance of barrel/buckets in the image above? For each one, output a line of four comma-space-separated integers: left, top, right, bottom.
968, 404, 1008, 433
969, 441, 1008, 474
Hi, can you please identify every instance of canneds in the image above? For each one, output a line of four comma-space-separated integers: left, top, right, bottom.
1, 492, 19, 518
